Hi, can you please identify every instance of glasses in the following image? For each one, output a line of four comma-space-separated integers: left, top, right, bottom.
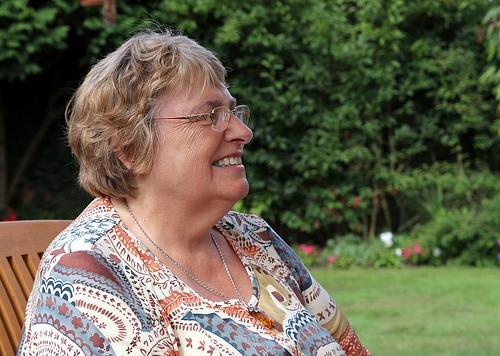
144, 103, 250, 132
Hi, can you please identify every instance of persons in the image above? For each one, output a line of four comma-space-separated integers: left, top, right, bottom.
15, 30, 373, 356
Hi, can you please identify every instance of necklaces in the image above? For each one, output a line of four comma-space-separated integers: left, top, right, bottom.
123, 196, 276, 330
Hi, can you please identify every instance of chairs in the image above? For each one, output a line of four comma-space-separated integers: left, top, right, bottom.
0, 220, 76, 356
